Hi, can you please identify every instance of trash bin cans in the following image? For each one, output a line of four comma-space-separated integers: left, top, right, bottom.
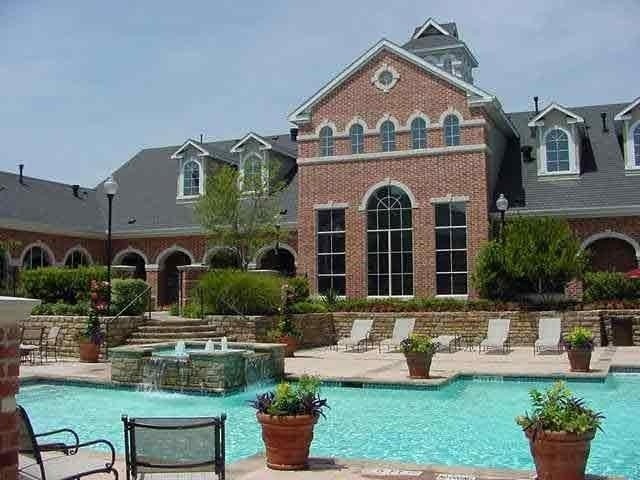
612, 317, 633, 346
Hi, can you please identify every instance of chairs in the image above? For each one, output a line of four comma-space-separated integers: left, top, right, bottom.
478, 318, 511, 354
378, 318, 416, 353
430, 335, 462, 352
336, 320, 375, 351
15, 404, 118, 480
121, 412, 227, 480
16, 323, 62, 366
532, 318, 562, 355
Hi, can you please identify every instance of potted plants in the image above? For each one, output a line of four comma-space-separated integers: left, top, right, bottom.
562, 324, 596, 371
399, 332, 435, 378
247, 376, 331, 470
278, 318, 304, 358
71, 321, 106, 363
514, 380, 604, 480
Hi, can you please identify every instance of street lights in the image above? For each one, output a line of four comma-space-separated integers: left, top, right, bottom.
496, 191, 509, 266
103, 174, 118, 363
9, 255, 23, 297
